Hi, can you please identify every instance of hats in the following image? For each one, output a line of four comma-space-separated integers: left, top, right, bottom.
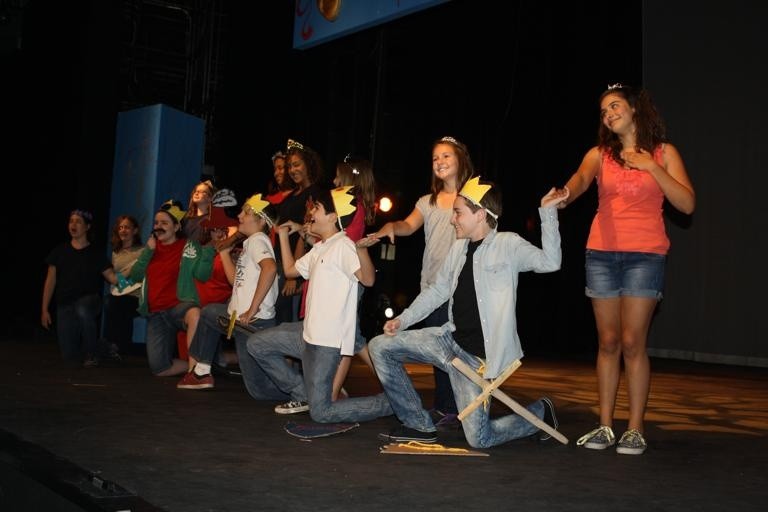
70, 209, 92, 218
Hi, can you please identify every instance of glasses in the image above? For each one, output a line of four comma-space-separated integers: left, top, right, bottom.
119, 225, 132, 230
194, 189, 211, 195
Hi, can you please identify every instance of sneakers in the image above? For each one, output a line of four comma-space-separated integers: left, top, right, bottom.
539, 397, 558, 441
378, 425, 438, 443
575, 426, 616, 450
336, 386, 348, 400
110, 350, 121, 360
615, 429, 648, 453
177, 366, 215, 389
275, 400, 309, 413
429, 408, 460, 425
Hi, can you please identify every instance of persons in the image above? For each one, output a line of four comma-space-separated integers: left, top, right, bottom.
181, 180, 215, 243
176, 190, 239, 366
176, 191, 292, 398
267, 139, 325, 366
213, 153, 296, 251
262, 150, 297, 235
246, 186, 391, 426
364, 137, 471, 429
368, 176, 569, 444
130, 200, 216, 375
279, 153, 377, 402
552, 84, 695, 455
41, 207, 135, 363
102, 215, 146, 364
